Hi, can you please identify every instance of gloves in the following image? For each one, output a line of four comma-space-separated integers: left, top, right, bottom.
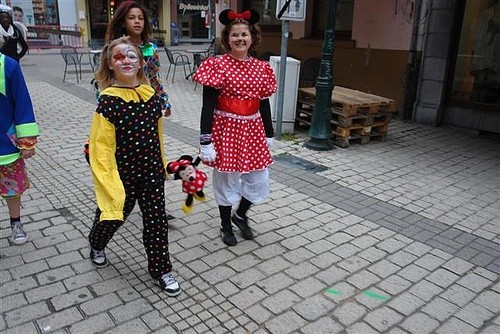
198, 142, 216, 162
265, 137, 273, 150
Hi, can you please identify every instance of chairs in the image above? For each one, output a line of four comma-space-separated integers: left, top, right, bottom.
61, 45, 97, 85
164, 46, 194, 83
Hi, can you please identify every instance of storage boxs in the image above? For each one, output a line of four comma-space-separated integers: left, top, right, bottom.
269, 56, 301, 135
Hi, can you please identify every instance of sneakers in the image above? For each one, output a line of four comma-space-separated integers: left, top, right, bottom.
10, 221, 28, 245
90, 244, 108, 268
150, 272, 182, 296
231, 212, 254, 240
220, 223, 237, 246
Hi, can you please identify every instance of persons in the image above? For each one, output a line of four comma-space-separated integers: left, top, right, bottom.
0, 33, 40, 245
13, 6, 24, 21
94, 1, 176, 223
0, 5, 29, 63
192, 9, 277, 246
84, 36, 182, 298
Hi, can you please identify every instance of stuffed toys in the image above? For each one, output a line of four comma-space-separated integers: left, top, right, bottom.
166, 155, 209, 215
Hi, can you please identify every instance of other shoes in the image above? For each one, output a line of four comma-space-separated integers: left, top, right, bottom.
165, 212, 175, 220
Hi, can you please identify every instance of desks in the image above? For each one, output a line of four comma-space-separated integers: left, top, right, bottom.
76, 49, 103, 80
185, 49, 210, 70
